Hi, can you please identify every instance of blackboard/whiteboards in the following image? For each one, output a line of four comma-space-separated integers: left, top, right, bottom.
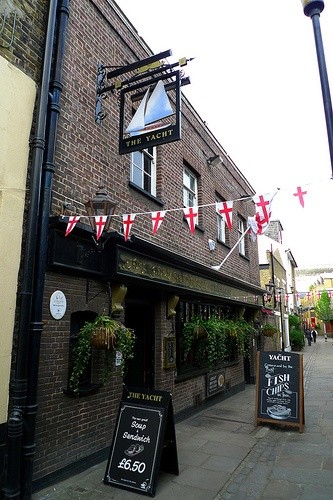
103, 386, 179, 497
255, 351, 303, 426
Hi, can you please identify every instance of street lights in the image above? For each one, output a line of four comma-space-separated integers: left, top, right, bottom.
302, 0, 333, 172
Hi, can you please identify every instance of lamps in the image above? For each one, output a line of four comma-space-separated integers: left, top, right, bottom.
240, 195, 252, 202
207, 155, 223, 166
75, 184, 119, 265
263, 278, 294, 309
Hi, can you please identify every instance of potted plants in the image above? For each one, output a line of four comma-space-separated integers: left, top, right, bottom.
183, 313, 280, 375
69, 315, 138, 397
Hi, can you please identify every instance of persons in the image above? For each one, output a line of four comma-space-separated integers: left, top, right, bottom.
305, 329, 312, 346
312, 331, 316, 343
